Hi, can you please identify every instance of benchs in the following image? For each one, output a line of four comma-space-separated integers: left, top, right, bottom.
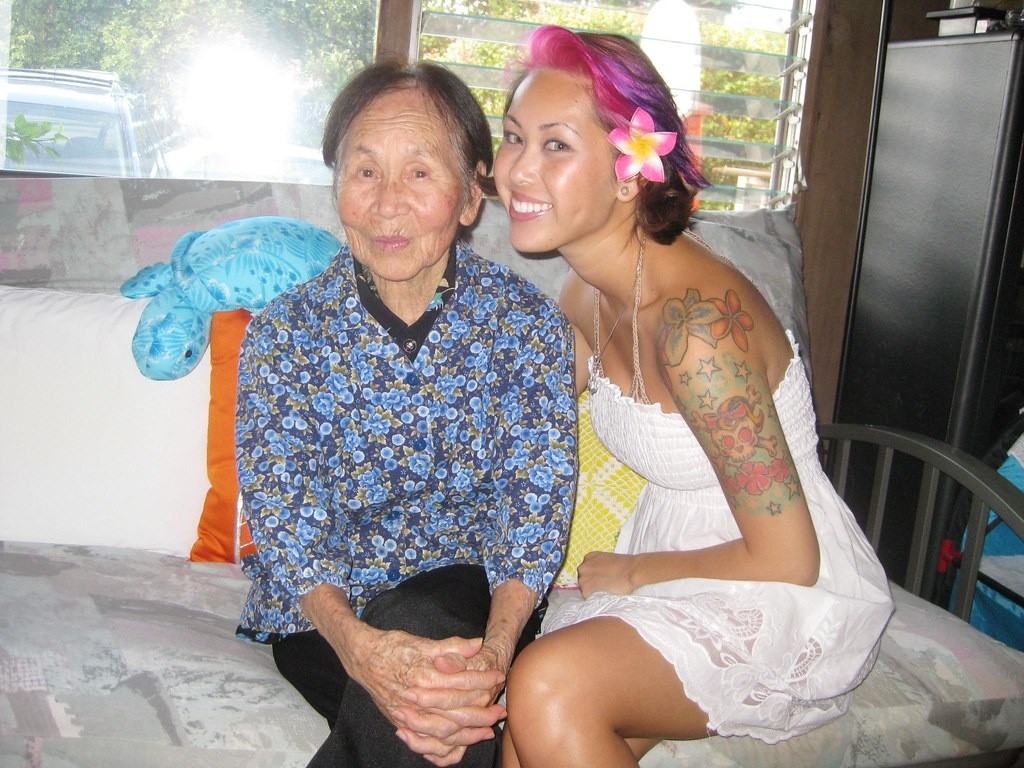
0, 170, 1024, 768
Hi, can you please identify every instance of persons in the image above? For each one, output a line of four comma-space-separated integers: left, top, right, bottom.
492, 24, 896, 768
236, 57, 580, 768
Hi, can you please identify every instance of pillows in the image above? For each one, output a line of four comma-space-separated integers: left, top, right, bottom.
0, 283, 213, 559
187, 308, 257, 563
554, 387, 646, 590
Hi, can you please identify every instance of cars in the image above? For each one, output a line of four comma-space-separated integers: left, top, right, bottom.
0, 64, 171, 183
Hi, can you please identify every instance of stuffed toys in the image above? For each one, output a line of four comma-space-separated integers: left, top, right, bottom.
120, 216, 343, 381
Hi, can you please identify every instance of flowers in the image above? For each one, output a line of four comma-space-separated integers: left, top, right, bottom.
605, 107, 677, 183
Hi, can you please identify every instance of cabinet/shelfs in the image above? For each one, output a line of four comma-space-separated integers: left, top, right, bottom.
827, 0, 1024, 597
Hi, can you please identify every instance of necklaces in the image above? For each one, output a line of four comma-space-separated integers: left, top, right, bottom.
587, 239, 644, 395
